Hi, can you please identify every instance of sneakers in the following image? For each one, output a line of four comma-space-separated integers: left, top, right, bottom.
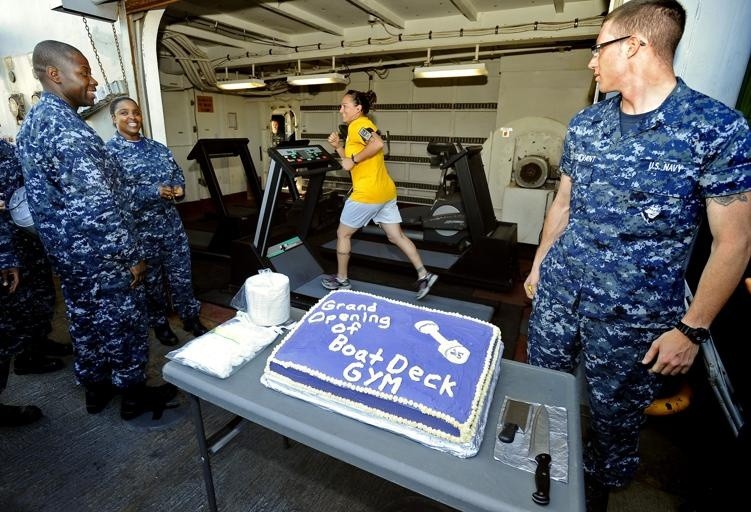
320, 276, 352, 289
414, 272, 439, 300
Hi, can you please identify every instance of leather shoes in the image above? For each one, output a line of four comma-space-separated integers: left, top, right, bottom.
0, 339, 73, 426
183, 317, 208, 337
154, 321, 179, 345
86, 379, 177, 420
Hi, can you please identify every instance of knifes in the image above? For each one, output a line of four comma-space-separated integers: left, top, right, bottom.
498, 398, 531, 443
527, 403, 553, 505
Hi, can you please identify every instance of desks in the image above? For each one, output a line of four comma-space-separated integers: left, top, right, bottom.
161, 304, 586, 511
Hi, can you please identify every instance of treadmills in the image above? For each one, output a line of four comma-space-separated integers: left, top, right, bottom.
229, 146, 497, 328
317, 145, 522, 295
170, 137, 266, 262
359, 140, 474, 251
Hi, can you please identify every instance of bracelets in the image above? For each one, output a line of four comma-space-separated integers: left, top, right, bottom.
350, 157, 359, 164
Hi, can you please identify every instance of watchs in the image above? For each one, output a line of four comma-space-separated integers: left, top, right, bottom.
675, 321, 713, 347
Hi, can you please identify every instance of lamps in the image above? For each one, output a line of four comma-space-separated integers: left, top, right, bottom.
214, 64, 267, 92
413, 44, 489, 81
287, 57, 350, 87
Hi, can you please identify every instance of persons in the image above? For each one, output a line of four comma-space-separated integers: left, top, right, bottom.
1, 194, 43, 426
16, 39, 179, 419
105, 97, 208, 347
321, 91, 440, 300
522, 0, 750, 512
1, 141, 74, 376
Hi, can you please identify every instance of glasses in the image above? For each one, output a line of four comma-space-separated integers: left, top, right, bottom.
590, 35, 645, 56
